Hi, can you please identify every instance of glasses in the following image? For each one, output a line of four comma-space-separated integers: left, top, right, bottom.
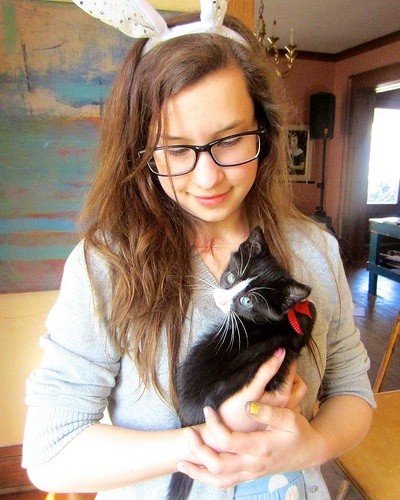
138, 128, 264, 177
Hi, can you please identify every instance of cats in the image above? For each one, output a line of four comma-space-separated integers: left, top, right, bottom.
178, 225, 316, 427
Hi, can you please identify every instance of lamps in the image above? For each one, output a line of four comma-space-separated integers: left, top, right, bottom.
251, 0, 297, 79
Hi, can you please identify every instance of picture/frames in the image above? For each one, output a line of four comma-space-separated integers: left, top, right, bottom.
279, 123, 311, 183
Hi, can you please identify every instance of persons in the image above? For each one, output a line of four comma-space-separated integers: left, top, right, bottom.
19, 9, 378, 500
285, 132, 305, 175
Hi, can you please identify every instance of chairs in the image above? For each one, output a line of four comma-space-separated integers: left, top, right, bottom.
311, 302, 400, 500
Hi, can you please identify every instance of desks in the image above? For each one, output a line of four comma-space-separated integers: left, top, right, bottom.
366, 216, 400, 297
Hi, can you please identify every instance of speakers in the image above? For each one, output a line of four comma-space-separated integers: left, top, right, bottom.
309, 92, 335, 139
